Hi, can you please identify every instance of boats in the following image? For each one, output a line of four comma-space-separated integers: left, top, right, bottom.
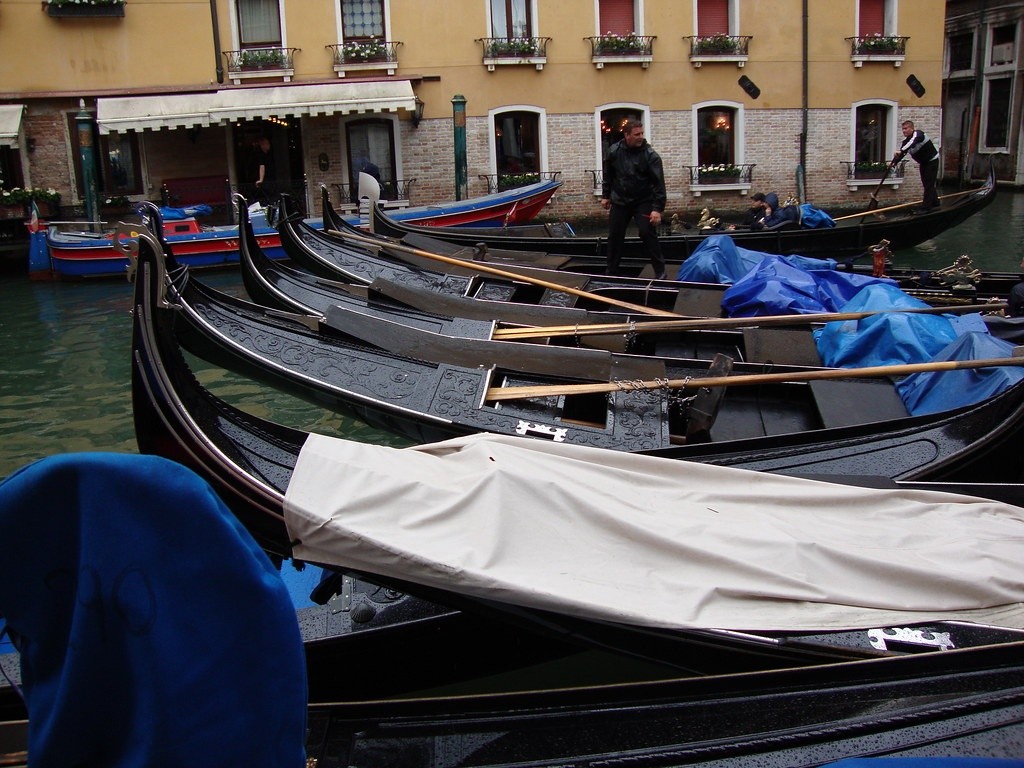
372, 149, 1002, 262
0, 182, 1022, 766
28, 178, 564, 286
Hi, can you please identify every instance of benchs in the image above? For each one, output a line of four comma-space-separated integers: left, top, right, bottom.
162, 176, 233, 225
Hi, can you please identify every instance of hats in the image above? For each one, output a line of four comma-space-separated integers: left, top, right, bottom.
751, 193, 765, 200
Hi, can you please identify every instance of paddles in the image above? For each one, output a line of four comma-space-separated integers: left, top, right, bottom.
833, 186, 991, 222
327, 229, 682, 317
859, 152, 900, 223
492, 303, 1009, 341
487, 354, 1024, 402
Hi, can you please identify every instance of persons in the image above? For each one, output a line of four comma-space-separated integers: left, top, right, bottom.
255, 137, 291, 222
602, 120, 668, 280
888, 121, 941, 214
728, 191, 789, 233
359, 157, 382, 185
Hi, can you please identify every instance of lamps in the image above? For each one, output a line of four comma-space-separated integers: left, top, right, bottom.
411, 94, 426, 129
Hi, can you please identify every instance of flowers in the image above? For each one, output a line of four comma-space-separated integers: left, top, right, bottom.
499, 172, 542, 188
698, 163, 740, 178
239, 47, 285, 65
855, 161, 893, 177
594, 31, 644, 52
0, 180, 61, 208
48, 0, 125, 8
857, 32, 899, 51
487, 39, 536, 54
95, 193, 129, 209
695, 32, 740, 50
343, 34, 388, 58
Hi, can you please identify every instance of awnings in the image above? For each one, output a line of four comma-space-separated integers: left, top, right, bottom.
0, 104, 28, 149
95, 80, 416, 136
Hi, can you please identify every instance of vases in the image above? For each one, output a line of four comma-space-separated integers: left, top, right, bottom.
700, 48, 735, 54
241, 64, 281, 71
48, 6, 125, 16
495, 52, 535, 57
859, 47, 900, 54
700, 177, 737, 184
855, 172, 883, 179
0, 204, 51, 220
600, 48, 639, 55
99, 204, 129, 214
343, 57, 387, 64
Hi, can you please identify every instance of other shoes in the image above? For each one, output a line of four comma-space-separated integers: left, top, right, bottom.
916, 208, 931, 215
930, 206, 941, 211
655, 272, 667, 280
604, 268, 621, 275
